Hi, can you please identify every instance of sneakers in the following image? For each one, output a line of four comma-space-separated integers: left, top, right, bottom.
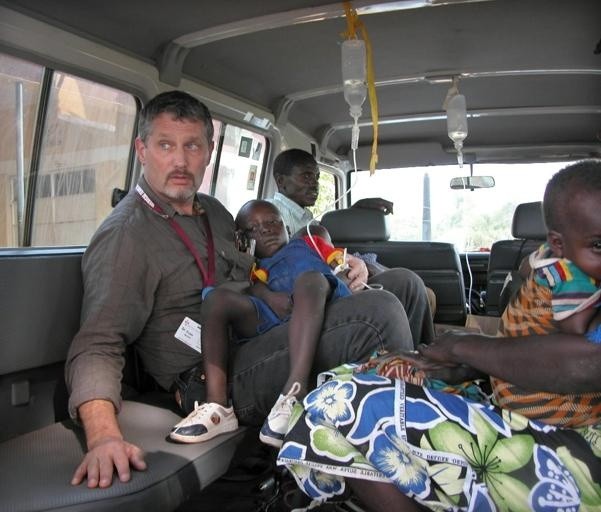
259, 394, 299, 448
171, 402, 239, 443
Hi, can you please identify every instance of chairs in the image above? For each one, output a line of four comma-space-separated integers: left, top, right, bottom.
484, 201, 548, 312
318, 202, 466, 326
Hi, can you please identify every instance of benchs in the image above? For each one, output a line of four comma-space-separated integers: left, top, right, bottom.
0, 245, 245, 512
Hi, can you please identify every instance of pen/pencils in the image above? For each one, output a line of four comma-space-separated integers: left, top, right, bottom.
247, 239, 256, 258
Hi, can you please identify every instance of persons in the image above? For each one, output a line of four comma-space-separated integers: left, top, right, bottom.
62, 90, 437, 489
274, 327, 601, 511
264, 148, 437, 324
167, 199, 370, 448
489, 160, 601, 429
